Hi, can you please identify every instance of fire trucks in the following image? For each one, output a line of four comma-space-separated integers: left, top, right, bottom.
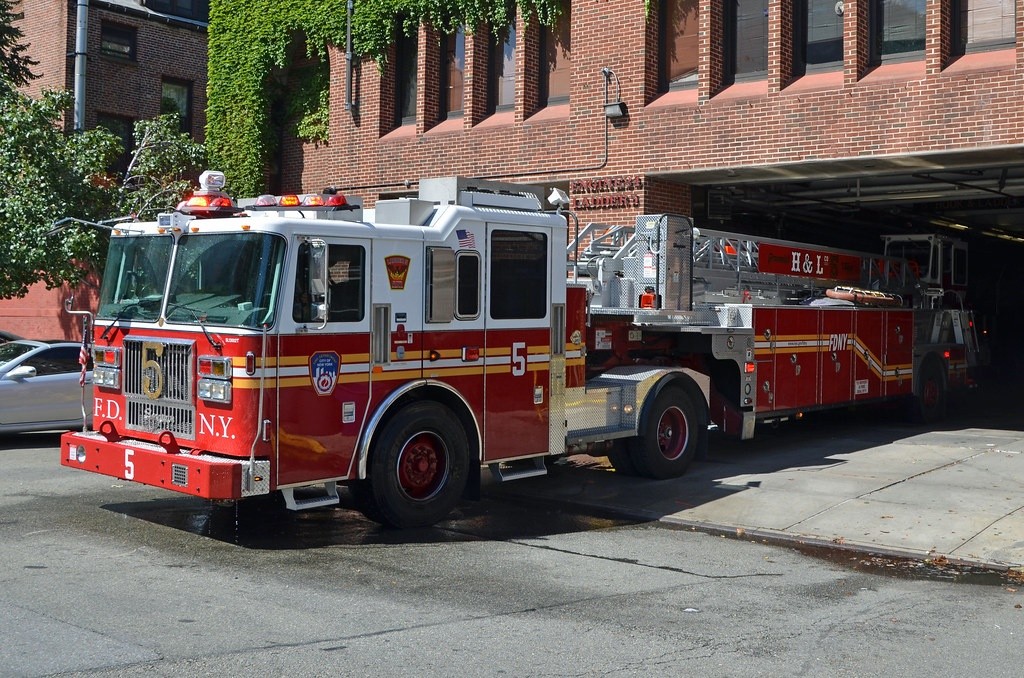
61, 169, 991, 532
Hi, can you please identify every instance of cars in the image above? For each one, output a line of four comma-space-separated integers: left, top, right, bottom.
0, 331, 93, 436
